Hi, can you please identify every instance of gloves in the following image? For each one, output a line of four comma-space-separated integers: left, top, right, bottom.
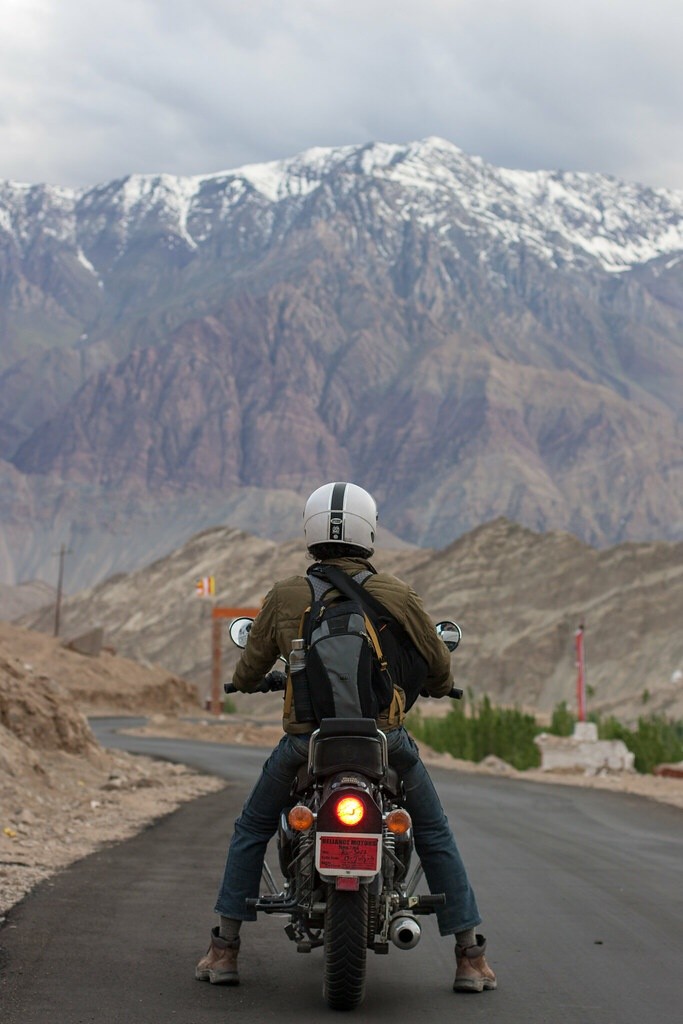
241, 676, 269, 693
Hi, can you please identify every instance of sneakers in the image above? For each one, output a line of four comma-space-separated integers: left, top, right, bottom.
195, 927, 241, 984
453, 934, 496, 991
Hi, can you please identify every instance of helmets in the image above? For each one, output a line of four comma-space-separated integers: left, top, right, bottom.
303, 482, 378, 558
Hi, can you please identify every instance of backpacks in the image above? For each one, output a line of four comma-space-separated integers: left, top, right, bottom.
289, 570, 395, 726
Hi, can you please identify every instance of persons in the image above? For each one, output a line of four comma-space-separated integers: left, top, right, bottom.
196, 482, 497, 992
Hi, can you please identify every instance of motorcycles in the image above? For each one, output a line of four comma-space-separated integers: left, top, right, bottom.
223, 616, 464, 1013
